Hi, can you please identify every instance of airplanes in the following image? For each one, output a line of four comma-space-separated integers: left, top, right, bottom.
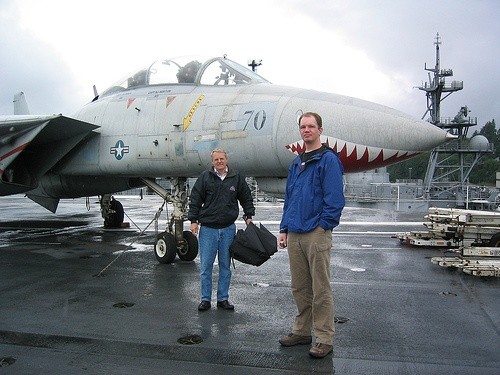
0, 53, 460, 264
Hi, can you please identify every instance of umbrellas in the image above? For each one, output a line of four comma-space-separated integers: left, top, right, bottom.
229, 215, 278, 269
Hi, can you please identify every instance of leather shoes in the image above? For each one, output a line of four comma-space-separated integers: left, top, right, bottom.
217, 300, 234, 309
198, 301, 210, 310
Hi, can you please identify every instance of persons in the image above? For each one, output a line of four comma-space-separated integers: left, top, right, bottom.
140, 188, 143, 200
279, 109, 345, 358
188, 147, 255, 311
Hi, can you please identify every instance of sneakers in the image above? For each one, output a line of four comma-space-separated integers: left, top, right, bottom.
279, 333, 312, 346
309, 344, 333, 357
